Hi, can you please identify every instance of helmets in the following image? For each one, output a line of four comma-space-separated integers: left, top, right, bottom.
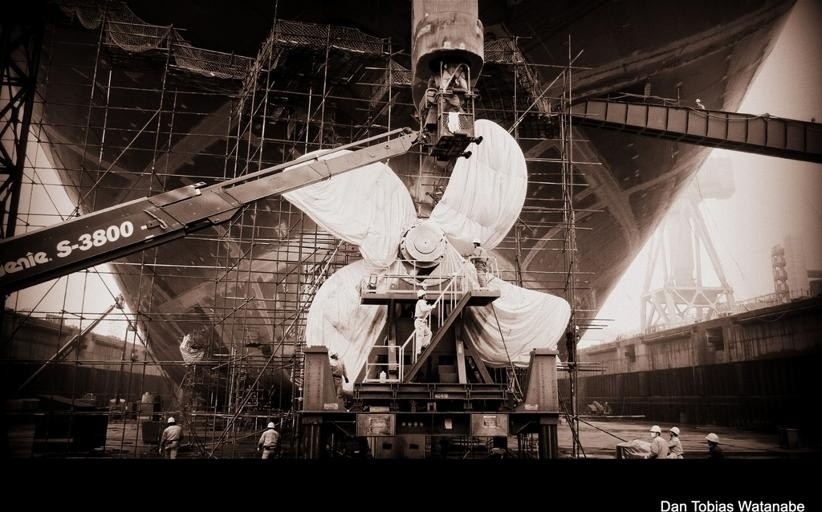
167, 417, 175, 424
649, 425, 662, 435
705, 433, 720, 444
473, 238, 481, 244
670, 427, 681, 436
267, 422, 275, 429
416, 290, 426, 298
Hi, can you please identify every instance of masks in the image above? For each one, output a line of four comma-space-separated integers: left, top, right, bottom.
650, 433, 659, 438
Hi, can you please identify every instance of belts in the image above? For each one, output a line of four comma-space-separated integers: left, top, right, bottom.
333, 375, 342, 378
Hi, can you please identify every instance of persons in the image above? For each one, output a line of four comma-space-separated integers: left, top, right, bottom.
693, 99, 705, 109
645, 424, 669, 460
705, 433, 724, 460
666, 427, 683, 459
257, 421, 280, 459
159, 416, 182, 459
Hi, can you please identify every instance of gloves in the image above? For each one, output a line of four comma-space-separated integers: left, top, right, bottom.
159, 449, 161, 455
